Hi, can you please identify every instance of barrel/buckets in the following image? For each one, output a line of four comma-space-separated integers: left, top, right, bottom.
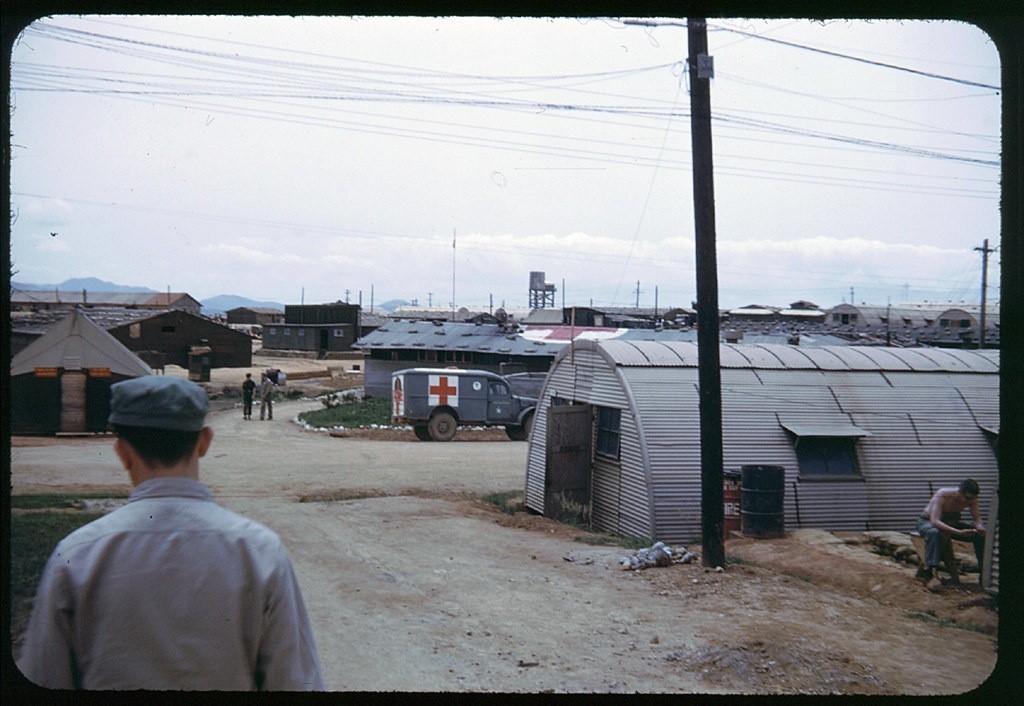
740, 465, 784, 538
722, 468, 740, 539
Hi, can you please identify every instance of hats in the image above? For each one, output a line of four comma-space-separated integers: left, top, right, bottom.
108, 374, 208, 431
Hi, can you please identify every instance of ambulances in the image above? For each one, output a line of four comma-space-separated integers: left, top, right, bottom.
391, 366, 539, 443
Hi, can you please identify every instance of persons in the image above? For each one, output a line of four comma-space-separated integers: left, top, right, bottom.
13, 375, 328, 692
241, 373, 257, 420
918, 480, 987, 593
259, 372, 274, 420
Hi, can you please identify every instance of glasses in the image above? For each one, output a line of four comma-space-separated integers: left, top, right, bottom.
964, 494, 973, 500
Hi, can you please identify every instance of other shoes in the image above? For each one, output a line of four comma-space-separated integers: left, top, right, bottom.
267, 417, 272, 420
922, 575, 944, 592
979, 573, 983, 584
260, 417, 264, 420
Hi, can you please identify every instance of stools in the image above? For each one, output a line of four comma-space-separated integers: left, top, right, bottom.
909, 531, 944, 583
